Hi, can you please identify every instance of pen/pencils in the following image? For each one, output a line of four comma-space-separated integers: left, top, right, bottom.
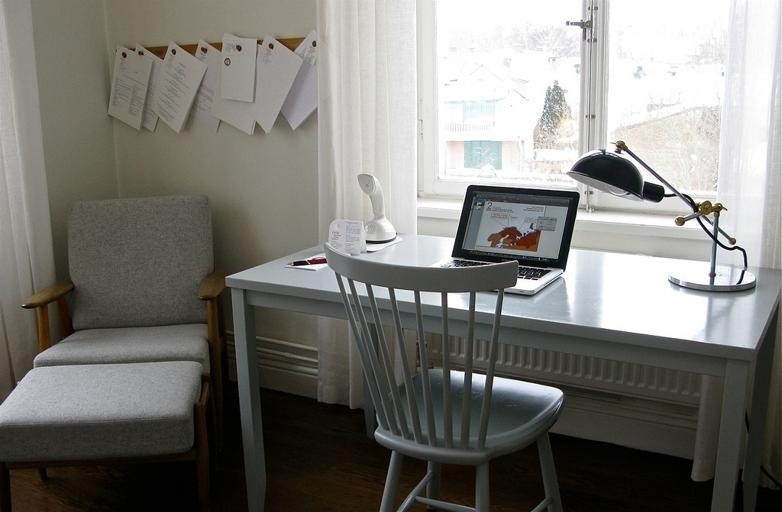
286, 258, 327, 267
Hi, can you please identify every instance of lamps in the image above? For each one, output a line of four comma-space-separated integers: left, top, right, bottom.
565, 140, 758, 293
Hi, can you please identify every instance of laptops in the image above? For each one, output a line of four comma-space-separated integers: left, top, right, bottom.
429, 184, 579, 295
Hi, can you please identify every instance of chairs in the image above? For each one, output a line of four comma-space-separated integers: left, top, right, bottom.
325, 243, 565, 512
0, 194, 229, 512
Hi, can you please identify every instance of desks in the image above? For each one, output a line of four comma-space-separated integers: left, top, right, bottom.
223, 230, 782, 512
